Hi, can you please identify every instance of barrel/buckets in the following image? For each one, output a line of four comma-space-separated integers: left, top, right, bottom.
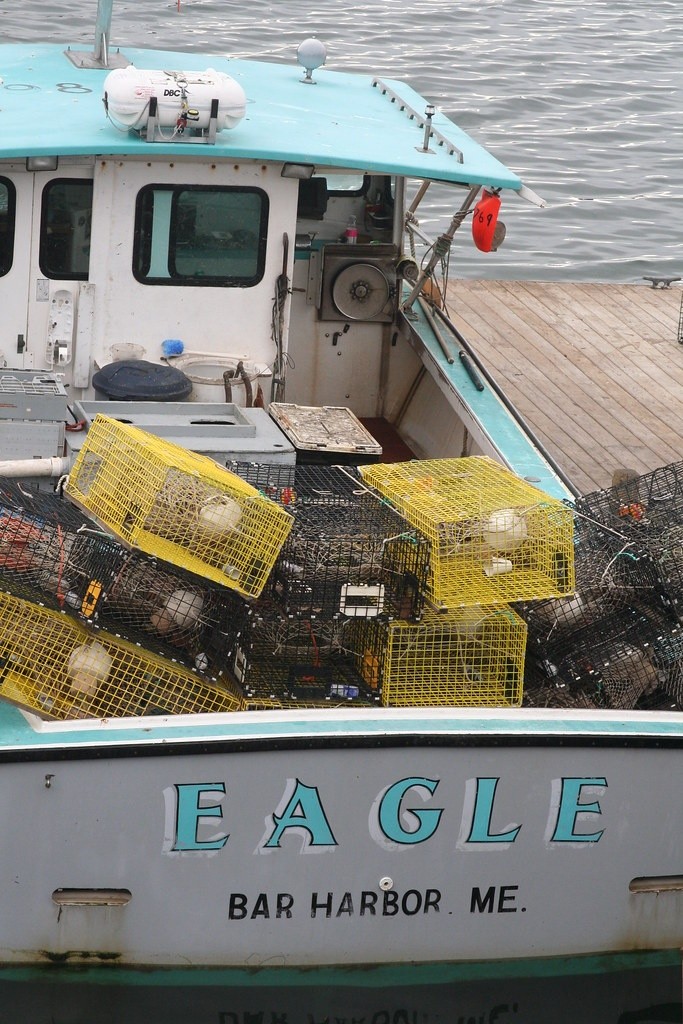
172, 354, 260, 409
92, 358, 192, 403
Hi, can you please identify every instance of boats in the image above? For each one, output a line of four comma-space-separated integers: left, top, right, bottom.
0, 40, 682, 1024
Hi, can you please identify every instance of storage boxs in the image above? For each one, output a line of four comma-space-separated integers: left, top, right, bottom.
267, 401, 383, 465
0, 412, 683, 725
